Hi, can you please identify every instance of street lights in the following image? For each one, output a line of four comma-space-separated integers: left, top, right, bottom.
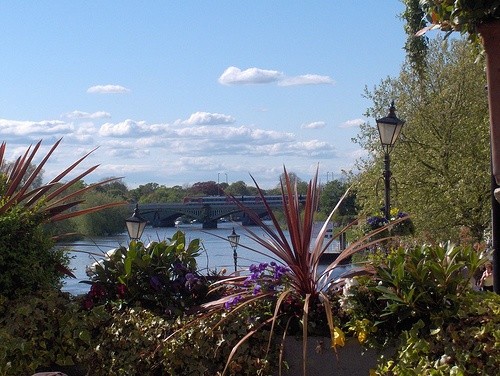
374, 97, 405, 221
227, 225, 240, 272
